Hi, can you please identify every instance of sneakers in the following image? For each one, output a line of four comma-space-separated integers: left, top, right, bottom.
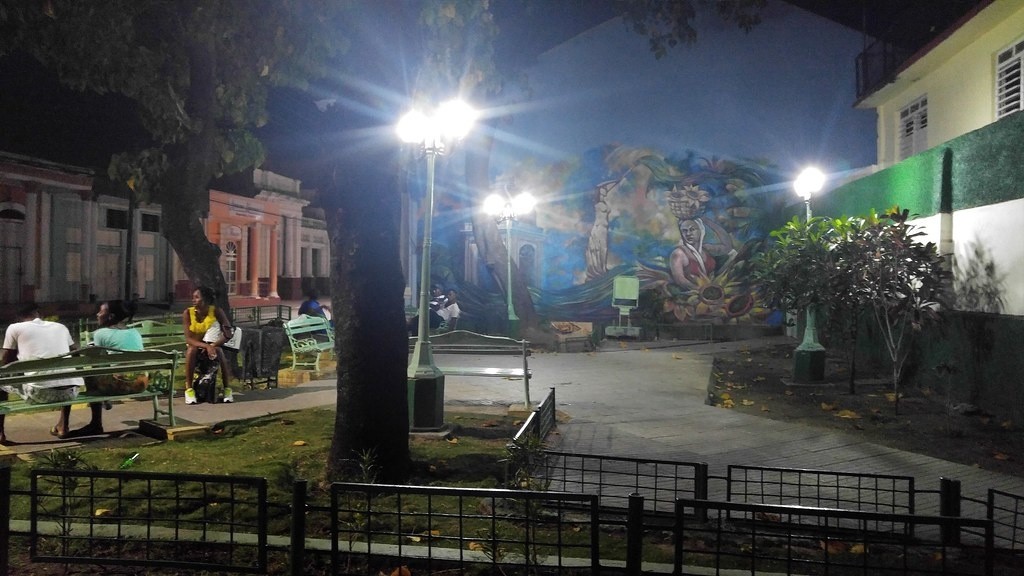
223, 388, 234, 403
184, 388, 198, 405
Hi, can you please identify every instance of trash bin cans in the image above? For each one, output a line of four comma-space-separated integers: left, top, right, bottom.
240, 324, 284, 378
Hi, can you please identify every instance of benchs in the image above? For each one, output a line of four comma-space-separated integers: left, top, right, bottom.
283, 314, 336, 371
415, 310, 459, 338
0, 348, 178, 428
80, 320, 224, 396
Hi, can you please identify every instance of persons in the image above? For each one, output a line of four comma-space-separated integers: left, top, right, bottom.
408, 283, 460, 336
298, 287, 334, 334
183, 286, 234, 405
74, 300, 148, 435
0, 300, 85, 445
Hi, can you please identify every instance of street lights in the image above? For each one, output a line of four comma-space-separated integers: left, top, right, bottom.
790, 166, 828, 383
395, 97, 479, 432
482, 184, 536, 334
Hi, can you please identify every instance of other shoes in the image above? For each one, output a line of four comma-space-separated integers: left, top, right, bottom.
80, 423, 104, 435
103, 400, 112, 411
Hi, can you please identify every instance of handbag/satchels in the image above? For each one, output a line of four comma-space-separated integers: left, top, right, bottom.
203, 322, 242, 353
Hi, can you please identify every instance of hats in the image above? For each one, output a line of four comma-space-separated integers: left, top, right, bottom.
434, 283, 444, 291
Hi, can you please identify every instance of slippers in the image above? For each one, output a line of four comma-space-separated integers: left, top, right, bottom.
50, 426, 69, 439
